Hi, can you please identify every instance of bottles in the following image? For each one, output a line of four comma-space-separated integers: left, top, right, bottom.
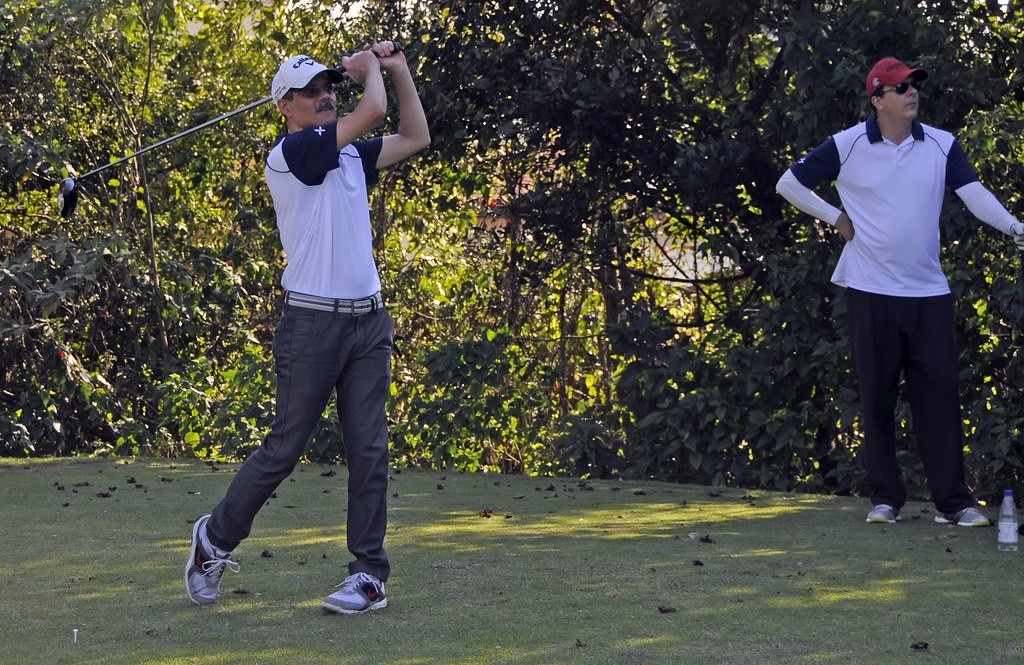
997, 490, 1018, 552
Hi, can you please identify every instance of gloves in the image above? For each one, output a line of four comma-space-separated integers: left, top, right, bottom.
1011, 223, 1024, 249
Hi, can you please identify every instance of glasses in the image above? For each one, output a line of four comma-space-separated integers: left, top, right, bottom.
287, 84, 335, 99
884, 79, 922, 95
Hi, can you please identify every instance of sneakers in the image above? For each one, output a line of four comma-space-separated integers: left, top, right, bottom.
322, 571, 388, 614
933, 507, 991, 527
867, 504, 902, 523
184, 516, 240, 606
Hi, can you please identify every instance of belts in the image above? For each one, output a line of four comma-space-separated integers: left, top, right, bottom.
283, 290, 384, 317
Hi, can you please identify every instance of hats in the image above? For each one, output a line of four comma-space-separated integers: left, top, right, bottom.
867, 58, 928, 98
272, 55, 345, 104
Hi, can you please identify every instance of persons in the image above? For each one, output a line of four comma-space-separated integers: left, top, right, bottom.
184, 41, 431, 615
777, 58, 1024, 525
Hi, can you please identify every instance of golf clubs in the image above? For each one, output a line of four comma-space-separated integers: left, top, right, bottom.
58, 41, 403, 220
